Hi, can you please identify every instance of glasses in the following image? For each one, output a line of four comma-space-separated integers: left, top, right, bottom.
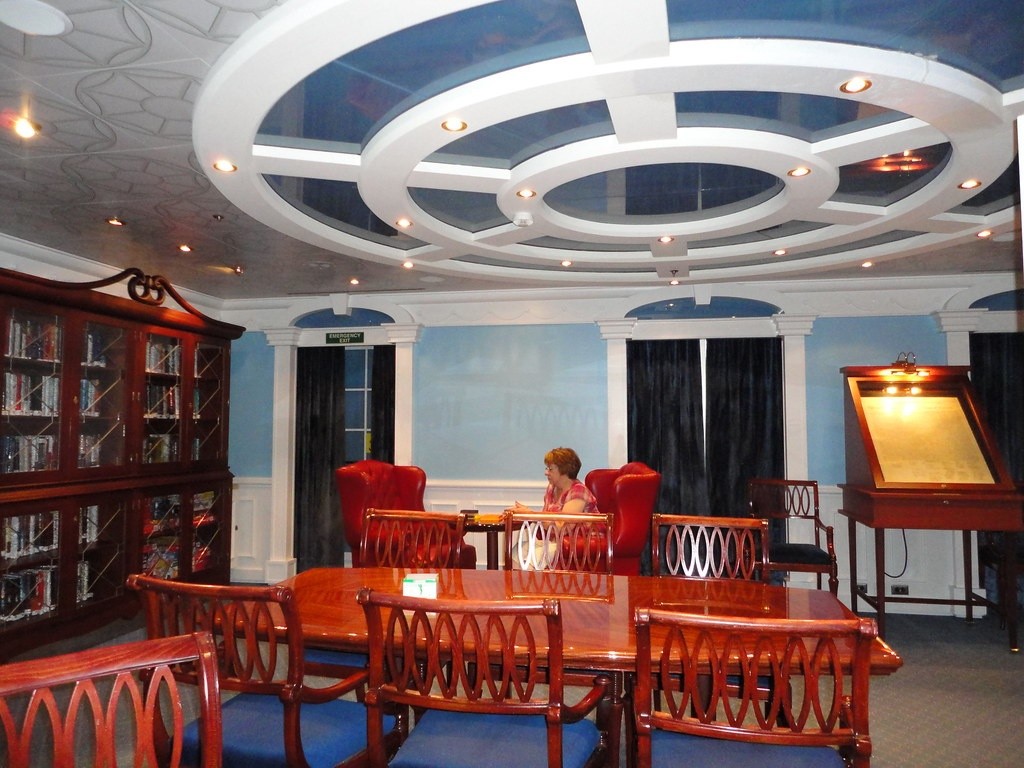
545, 466, 557, 471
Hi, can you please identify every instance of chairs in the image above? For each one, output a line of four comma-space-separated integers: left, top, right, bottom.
747, 478, 839, 598
0, 505, 878, 768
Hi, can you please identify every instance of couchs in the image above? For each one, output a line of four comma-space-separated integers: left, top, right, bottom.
546, 463, 661, 576
336, 460, 477, 570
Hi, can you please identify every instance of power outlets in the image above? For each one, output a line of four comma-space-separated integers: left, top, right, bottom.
891, 585, 908, 595
856, 584, 867, 593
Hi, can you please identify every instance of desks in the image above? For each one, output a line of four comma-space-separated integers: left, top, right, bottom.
197, 568, 903, 768
448, 513, 533, 570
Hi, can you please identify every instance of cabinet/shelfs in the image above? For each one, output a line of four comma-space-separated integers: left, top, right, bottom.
0, 268, 235, 665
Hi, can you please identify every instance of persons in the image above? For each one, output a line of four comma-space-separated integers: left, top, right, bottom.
504, 447, 605, 571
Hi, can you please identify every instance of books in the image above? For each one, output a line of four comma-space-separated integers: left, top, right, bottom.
0, 506, 100, 625
142, 490, 215, 583
0, 317, 106, 474
142, 342, 200, 463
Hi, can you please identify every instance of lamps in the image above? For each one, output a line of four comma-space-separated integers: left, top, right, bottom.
892, 352, 930, 376
232, 263, 247, 276
